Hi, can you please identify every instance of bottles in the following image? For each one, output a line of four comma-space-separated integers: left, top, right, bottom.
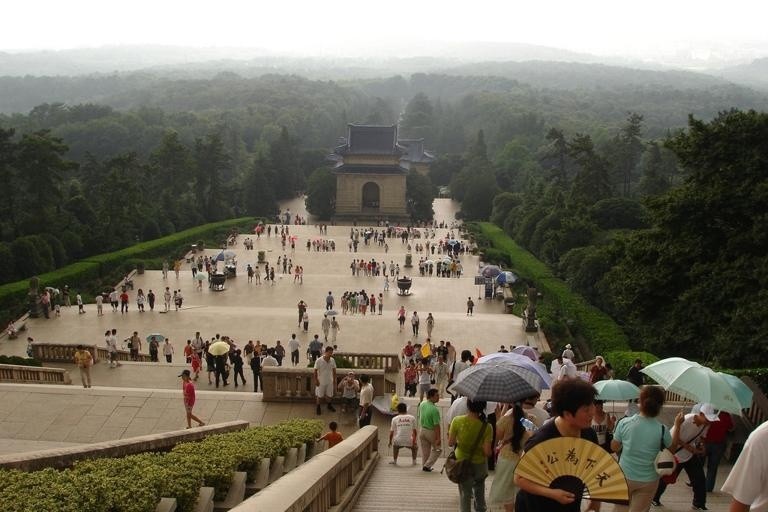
699, 443, 705, 457
520, 418, 539, 432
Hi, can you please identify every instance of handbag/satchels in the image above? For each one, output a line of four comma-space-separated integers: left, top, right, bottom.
84, 352, 93, 367
446, 361, 457, 395
440, 419, 488, 484
655, 424, 678, 477
429, 373, 435, 385
127, 336, 133, 348
661, 424, 708, 484
494, 435, 515, 465
606, 428, 614, 454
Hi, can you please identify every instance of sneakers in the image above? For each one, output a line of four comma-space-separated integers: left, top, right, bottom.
650, 499, 663, 508
317, 408, 321, 415
326, 405, 336, 412
706, 489, 713, 493
685, 482, 692, 488
186, 426, 192, 430
388, 462, 397, 465
197, 423, 205, 427
413, 462, 421, 465
689, 504, 711, 511
699, 404, 721, 422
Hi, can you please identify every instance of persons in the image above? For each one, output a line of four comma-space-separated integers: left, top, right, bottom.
448, 399, 494, 511
234, 349, 246, 387
215, 355, 230, 388
315, 223, 328, 235
285, 209, 290, 225
340, 289, 369, 315
256, 225, 260, 237
313, 238, 336, 252
247, 238, 250, 250
322, 314, 330, 342
591, 399, 616, 454
26, 336, 34, 357
105, 330, 111, 363
415, 243, 419, 254
199, 257, 203, 271
413, 344, 423, 365
271, 348, 280, 365
522, 391, 550, 428
294, 266, 300, 283
650, 404, 720, 511
204, 256, 208, 271
302, 309, 309, 332
333, 345, 338, 353
277, 256, 281, 273
286, 226, 289, 236
370, 294, 376, 315
267, 225, 271, 236
148, 289, 155, 310
109, 288, 118, 313
378, 294, 383, 315
291, 238, 295, 252
468, 355, 475, 366
136, 292, 145, 313
250, 239, 253, 250
294, 214, 306, 225
356, 259, 361, 276
184, 340, 193, 363
326, 291, 334, 310
275, 341, 285, 366
427, 318, 433, 337
177, 289, 184, 308
309, 335, 321, 363
8, 320, 16, 338
411, 311, 420, 337
425, 312, 434, 326
164, 287, 171, 312
163, 259, 169, 278
124, 273, 133, 290
264, 262, 269, 280
260, 224, 264, 234
512, 379, 601, 511
191, 332, 204, 371
280, 224, 284, 234
720, 373, 768, 511
393, 264, 399, 282
347, 225, 388, 255
384, 275, 389, 291
76, 291, 86, 314
41, 292, 50, 319
416, 388, 442, 472
535, 357, 546, 371
254, 266, 262, 285
163, 338, 175, 363
359, 374, 375, 428
338, 371, 359, 413
448, 351, 471, 405
260, 349, 278, 367
510, 345, 515, 350
446, 342, 456, 369
408, 244, 411, 254
124, 331, 141, 361
589, 358, 607, 382
467, 297, 474, 316
247, 264, 253, 283
376, 263, 380, 276
398, 306, 406, 332
298, 300, 308, 326
282, 233, 286, 241
603, 363, 613, 380
197, 257, 200, 269
558, 357, 568, 381
275, 226, 278, 234
251, 351, 263, 393
533, 346, 541, 358
364, 263, 367, 275
62, 285, 71, 307
96, 292, 104, 315
403, 341, 415, 368
270, 267, 276, 287
283, 255, 288, 273
207, 264, 212, 282
306, 345, 311, 360
191, 260, 197, 278
367, 261, 371, 276
255, 340, 262, 356
49, 289, 54, 310
288, 259, 292, 274
611, 386, 684, 511
627, 360, 644, 402
172, 291, 180, 311
244, 239, 247, 250
389, 225, 421, 244
421, 338, 433, 360
447, 393, 470, 437
120, 288, 129, 314
261, 344, 267, 358
388, 403, 420, 466
414, 359, 434, 406
244, 340, 254, 365
197, 280, 202, 291
404, 360, 419, 397
138, 288, 145, 300
432, 356, 449, 399
206, 350, 216, 385
54, 287, 61, 306
288, 334, 301, 366
110, 329, 121, 368
55, 303, 61, 317
371, 259, 376, 276
316, 422, 343, 448
232, 258, 235, 265
229, 236, 235, 243
360, 259, 364, 276
436, 340, 448, 363
205, 333, 236, 371
488, 401, 535, 511
420, 244, 423, 254
178, 370, 205, 429
382, 261, 386, 275
331, 316, 340, 342
314, 346, 337, 415
300, 265, 304, 285
189, 349, 200, 381
351, 259, 355, 276
149, 337, 160, 362
282, 239, 286, 251
418, 256, 463, 278
498, 346, 506, 352
390, 260, 395, 276
686, 409, 733, 493
75, 344, 94, 388
422, 221, 475, 259
307, 239, 311, 252
321, 343, 325, 356
175, 258, 181, 279
562, 344, 575, 364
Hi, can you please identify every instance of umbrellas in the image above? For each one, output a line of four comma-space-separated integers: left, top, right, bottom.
208, 340, 230, 356
640, 357, 742, 416
212, 250, 237, 262
146, 334, 164, 343
478, 353, 553, 390
511, 345, 539, 362
496, 272, 518, 285
718, 373, 753, 409
448, 359, 542, 402
593, 380, 642, 400
481, 265, 501, 277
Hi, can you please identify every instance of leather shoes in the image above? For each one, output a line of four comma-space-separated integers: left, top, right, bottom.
423, 466, 432, 472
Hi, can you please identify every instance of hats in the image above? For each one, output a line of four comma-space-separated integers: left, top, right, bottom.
177, 370, 192, 380
566, 343, 572, 349
